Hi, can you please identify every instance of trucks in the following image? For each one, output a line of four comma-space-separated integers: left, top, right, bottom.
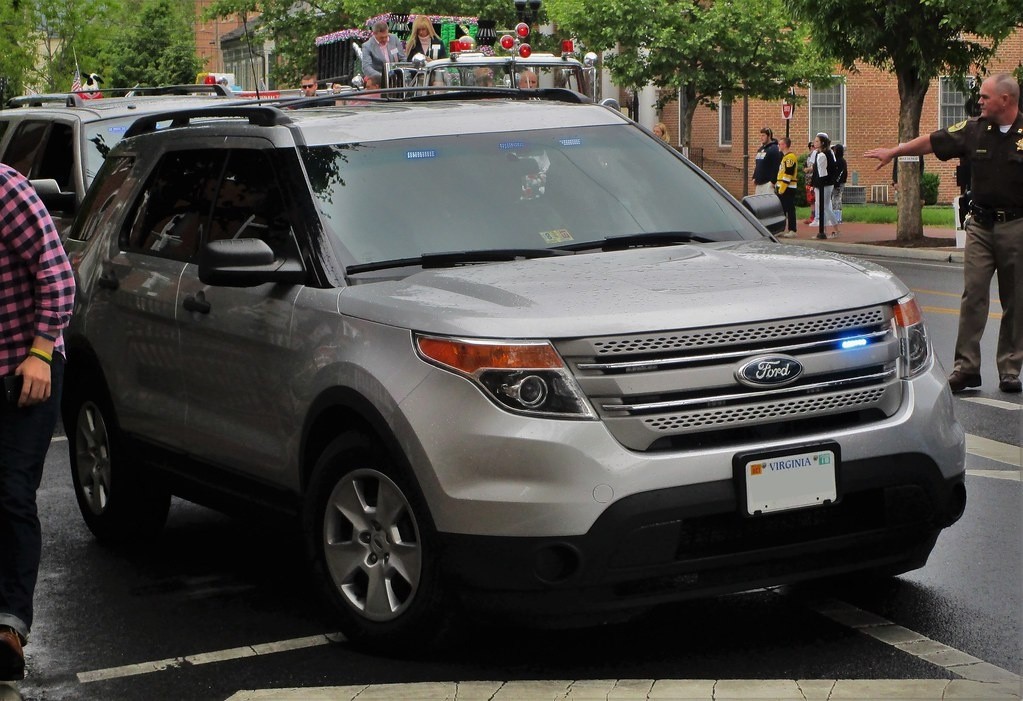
379, 19, 601, 104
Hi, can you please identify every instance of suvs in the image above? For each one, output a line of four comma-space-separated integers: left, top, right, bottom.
58, 85, 971, 662
0, 79, 282, 260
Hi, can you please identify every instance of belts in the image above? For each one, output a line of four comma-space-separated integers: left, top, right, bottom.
970, 201, 1023, 223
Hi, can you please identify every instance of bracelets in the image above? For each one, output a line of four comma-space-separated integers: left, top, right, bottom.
28, 347, 53, 368
34, 329, 57, 344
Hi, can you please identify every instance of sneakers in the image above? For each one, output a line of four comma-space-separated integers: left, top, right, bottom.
999, 373, 1022, 392
949, 371, 981, 391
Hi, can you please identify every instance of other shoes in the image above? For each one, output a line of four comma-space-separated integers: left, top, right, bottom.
827, 231, 841, 238
784, 230, 796, 238
804, 220, 812, 224
0, 624, 25, 681
809, 219, 819, 226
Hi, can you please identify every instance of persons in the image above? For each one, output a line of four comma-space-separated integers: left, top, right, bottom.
330, 81, 348, 107
775, 137, 799, 238
361, 20, 407, 97
651, 123, 670, 146
752, 128, 782, 197
300, 74, 320, 98
803, 131, 848, 239
0, 162, 78, 681
277, 84, 298, 90
408, 16, 448, 83
212, 76, 229, 96
352, 75, 381, 106
863, 74, 1023, 393
517, 71, 538, 90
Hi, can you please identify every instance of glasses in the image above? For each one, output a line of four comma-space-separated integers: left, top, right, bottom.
302, 82, 316, 89
809, 144, 814, 148
